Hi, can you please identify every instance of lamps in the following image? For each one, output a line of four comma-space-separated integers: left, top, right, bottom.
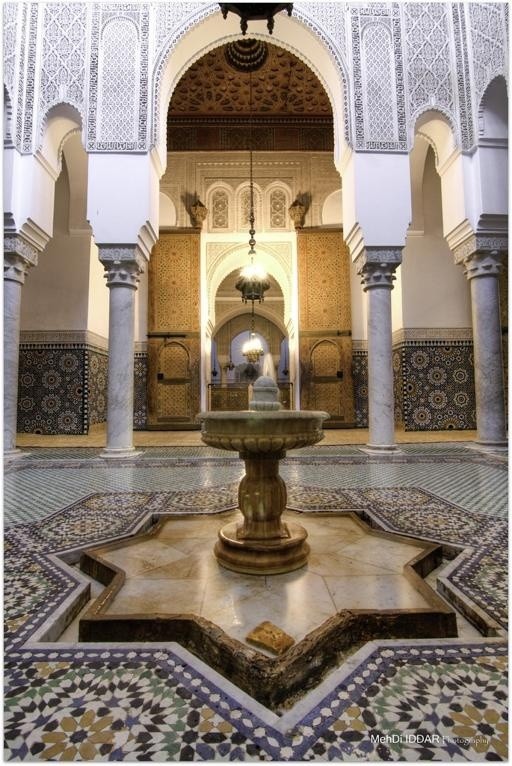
234, 38, 272, 332
241, 298, 265, 380
